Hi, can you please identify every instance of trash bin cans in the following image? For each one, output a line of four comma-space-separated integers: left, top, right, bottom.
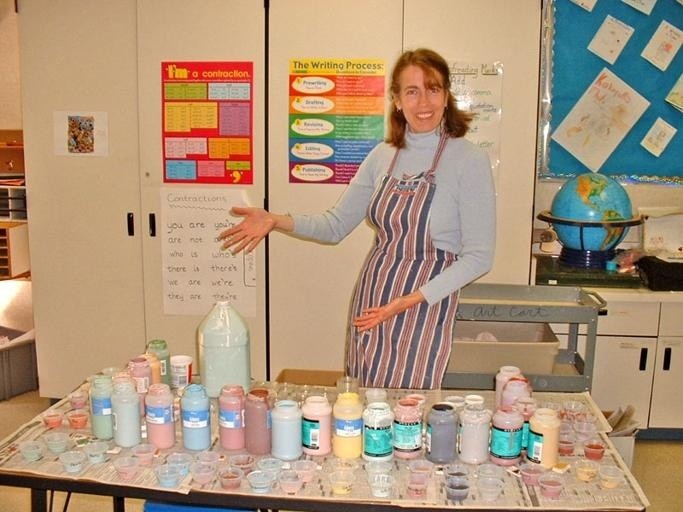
601, 410, 634, 470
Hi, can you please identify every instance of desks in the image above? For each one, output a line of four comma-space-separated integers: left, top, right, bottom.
0, 371, 656, 512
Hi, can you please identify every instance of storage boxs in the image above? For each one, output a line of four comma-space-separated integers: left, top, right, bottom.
609, 436, 634, 470
0, 337, 38, 400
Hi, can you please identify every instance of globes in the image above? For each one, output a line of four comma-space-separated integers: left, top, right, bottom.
536, 173, 643, 270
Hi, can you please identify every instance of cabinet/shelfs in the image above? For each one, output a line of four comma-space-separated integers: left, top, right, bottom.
0, 144, 31, 278
435, 285, 606, 396
546, 289, 683, 441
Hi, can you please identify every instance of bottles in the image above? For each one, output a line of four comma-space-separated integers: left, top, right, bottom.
332, 392, 362, 456
424, 403, 457, 465
512, 396, 539, 455
178, 384, 211, 453
217, 383, 245, 450
270, 399, 304, 461
457, 394, 491, 464
391, 397, 424, 462
85, 340, 177, 450
362, 403, 394, 461
190, 452, 569, 504
245, 362, 560, 417
301, 395, 332, 457
245, 388, 279, 455
525, 410, 558, 470
489, 406, 523, 465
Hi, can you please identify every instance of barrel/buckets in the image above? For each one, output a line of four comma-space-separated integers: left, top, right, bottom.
196, 297, 251, 399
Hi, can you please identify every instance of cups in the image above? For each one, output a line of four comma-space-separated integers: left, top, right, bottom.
19, 430, 191, 489
169, 356, 193, 388
543, 398, 623, 491
42, 383, 88, 430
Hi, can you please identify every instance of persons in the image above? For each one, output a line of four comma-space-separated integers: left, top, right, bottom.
217, 48, 498, 390
